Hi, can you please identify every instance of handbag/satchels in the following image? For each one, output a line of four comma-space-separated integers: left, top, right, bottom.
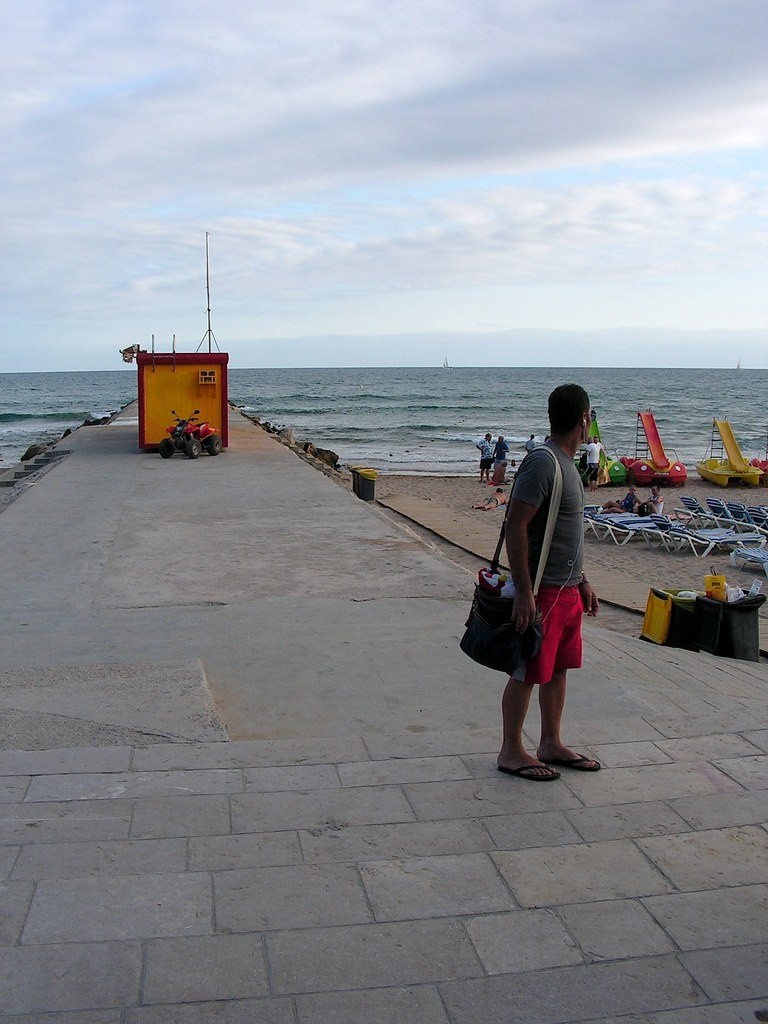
638, 503, 648, 517
459, 582, 543, 682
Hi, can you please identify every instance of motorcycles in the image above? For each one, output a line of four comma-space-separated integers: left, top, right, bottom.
159, 410, 222, 459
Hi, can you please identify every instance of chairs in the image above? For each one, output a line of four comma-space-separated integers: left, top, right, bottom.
628, 459, 640, 465
584, 497, 768, 576
705, 459, 719, 470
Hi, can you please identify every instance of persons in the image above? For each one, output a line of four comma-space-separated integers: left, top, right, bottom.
471, 488, 508, 511
492, 436, 515, 484
586, 435, 603, 492
632, 484, 664, 515
496, 383, 601, 780
526, 434, 536, 453
476, 433, 494, 483
589, 485, 642, 514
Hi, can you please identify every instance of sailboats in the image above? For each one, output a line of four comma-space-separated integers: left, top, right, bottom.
443, 358, 455, 373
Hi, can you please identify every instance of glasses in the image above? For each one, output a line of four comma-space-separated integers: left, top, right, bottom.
586, 410, 596, 421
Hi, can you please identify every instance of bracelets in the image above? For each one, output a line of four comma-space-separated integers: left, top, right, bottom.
579, 579, 590, 587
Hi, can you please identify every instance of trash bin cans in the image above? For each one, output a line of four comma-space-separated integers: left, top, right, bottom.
350, 467, 376, 500
642, 566, 766, 660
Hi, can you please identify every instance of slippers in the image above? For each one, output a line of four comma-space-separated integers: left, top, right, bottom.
538, 753, 600, 771
497, 762, 561, 781
